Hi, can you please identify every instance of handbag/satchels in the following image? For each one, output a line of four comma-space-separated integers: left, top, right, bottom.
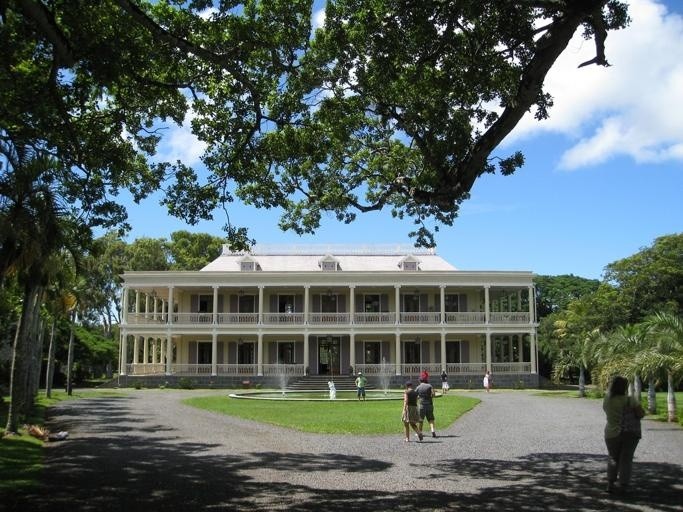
620, 407, 641, 441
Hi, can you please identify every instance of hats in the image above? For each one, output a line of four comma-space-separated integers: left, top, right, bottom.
406, 382, 412, 385
358, 372, 362, 374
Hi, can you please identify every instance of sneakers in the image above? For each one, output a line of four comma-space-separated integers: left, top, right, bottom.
404, 431, 436, 442
606, 484, 614, 492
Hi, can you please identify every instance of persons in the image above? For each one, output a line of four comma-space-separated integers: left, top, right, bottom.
354, 371, 369, 401
482, 370, 492, 393
414, 376, 437, 437
440, 371, 448, 394
400, 381, 424, 443
601, 375, 646, 494
419, 370, 428, 379
